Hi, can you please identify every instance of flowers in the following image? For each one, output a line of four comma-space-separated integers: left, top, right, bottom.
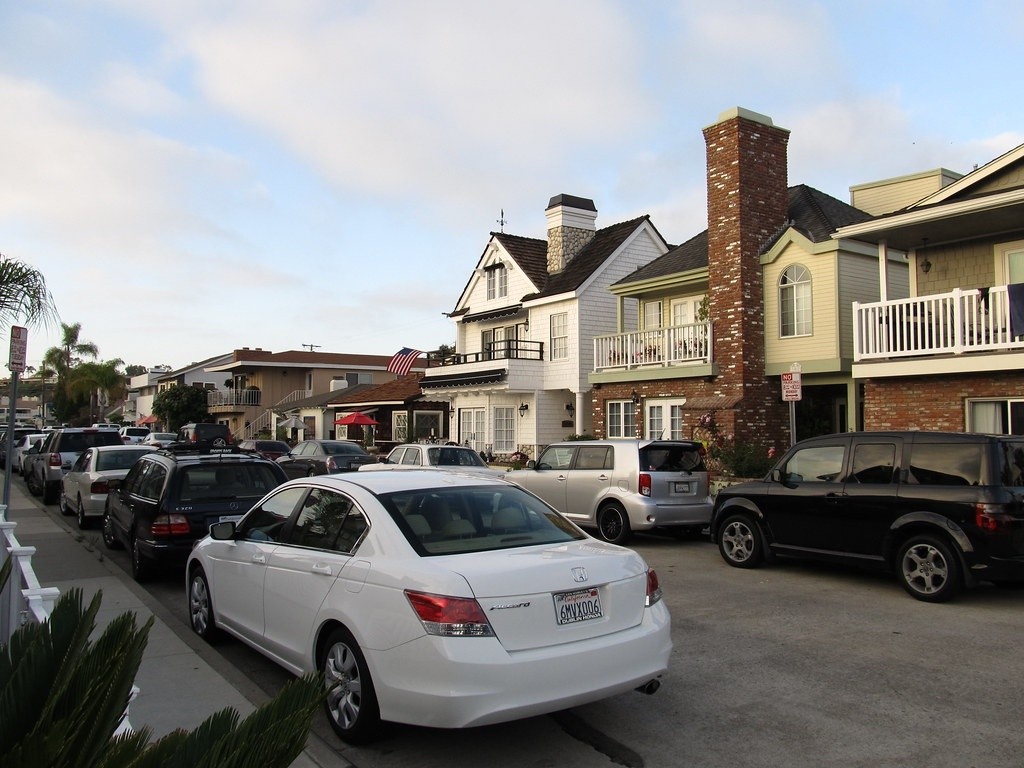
697, 412, 730, 471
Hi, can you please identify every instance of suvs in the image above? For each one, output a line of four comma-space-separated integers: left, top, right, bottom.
118, 425, 151, 444
103, 448, 291, 588
710, 424, 1023, 602
25, 427, 124, 506
165, 423, 235, 453
0, 428, 44, 472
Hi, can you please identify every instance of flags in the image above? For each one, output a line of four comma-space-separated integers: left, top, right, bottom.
386, 347, 425, 377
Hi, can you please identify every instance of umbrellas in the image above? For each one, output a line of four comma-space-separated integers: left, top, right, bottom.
276, 414, 311, 442
331, 411, 383, 445
134, 413, 164, 435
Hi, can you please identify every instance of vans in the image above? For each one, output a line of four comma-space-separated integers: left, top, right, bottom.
500, 428, 714, 543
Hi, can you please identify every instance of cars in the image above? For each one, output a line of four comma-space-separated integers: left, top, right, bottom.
141, 431, 178, 452
67, 444, 161, 533
357, 444, 509, 482
237, 439, 291, 460
180, 467, 674, 747
22, 438, 45, 480
12, 433, 48, 474
274, 438, 379, 480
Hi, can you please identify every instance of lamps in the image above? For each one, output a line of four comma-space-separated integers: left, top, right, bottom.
631, 389, 636, 401
524, 319, 529, 331
519, 402, 525, 418
920, 238, 931, 273
569, 403, 574, 417
449, 408, 454, 418
